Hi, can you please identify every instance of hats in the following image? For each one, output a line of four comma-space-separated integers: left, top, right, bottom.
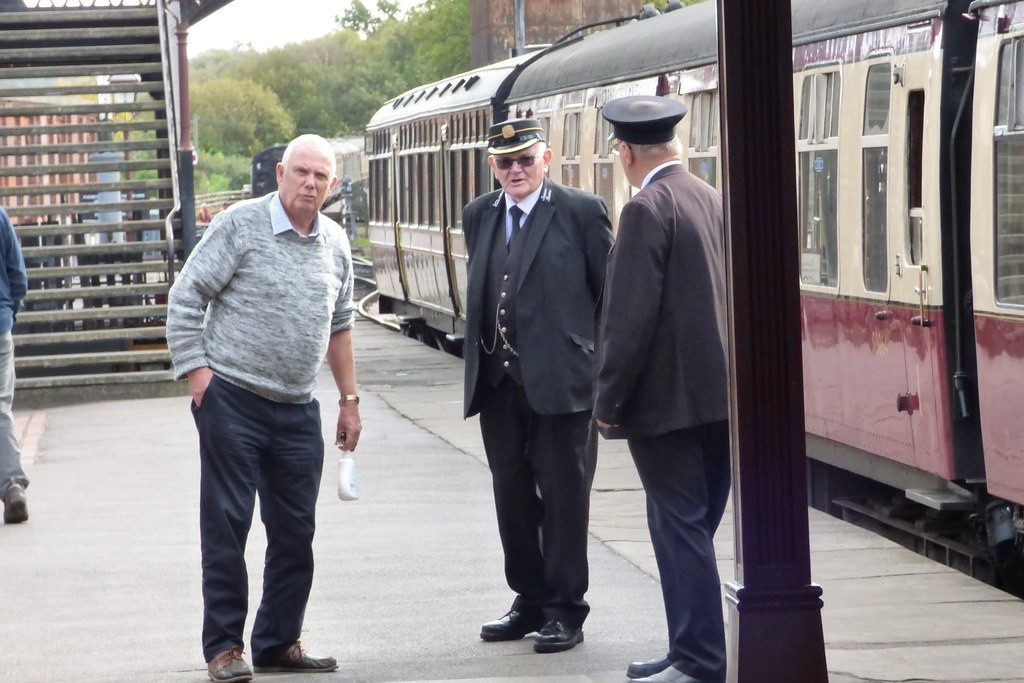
601, 96, 688, 145
487, 119, 546, 155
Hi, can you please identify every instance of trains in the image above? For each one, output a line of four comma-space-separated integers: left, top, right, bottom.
251, 136, 365, 210
365, 2, 1024, 578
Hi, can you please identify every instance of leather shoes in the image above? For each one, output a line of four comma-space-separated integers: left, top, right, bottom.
534, 619, 584, 654
626, 653, 708, 683
207, 645, 254, 683
254, 641, 339, 672
480, 609, 553, 642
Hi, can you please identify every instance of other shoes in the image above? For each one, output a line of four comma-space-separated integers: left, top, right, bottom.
3, 475, 29, 525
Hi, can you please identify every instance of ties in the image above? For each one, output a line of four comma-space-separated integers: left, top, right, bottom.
507, 206, 524, 255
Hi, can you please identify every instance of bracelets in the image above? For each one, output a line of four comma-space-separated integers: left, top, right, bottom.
339, 395, 360, 405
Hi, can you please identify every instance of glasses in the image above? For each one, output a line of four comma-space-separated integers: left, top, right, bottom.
610, 138, 625, 156
495, 152, 542, 170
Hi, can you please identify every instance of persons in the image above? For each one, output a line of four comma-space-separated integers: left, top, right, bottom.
464, 120, 615, 654
166, 134, 362, 683
594, 95, 731, 683
0, 205, 33, 523
